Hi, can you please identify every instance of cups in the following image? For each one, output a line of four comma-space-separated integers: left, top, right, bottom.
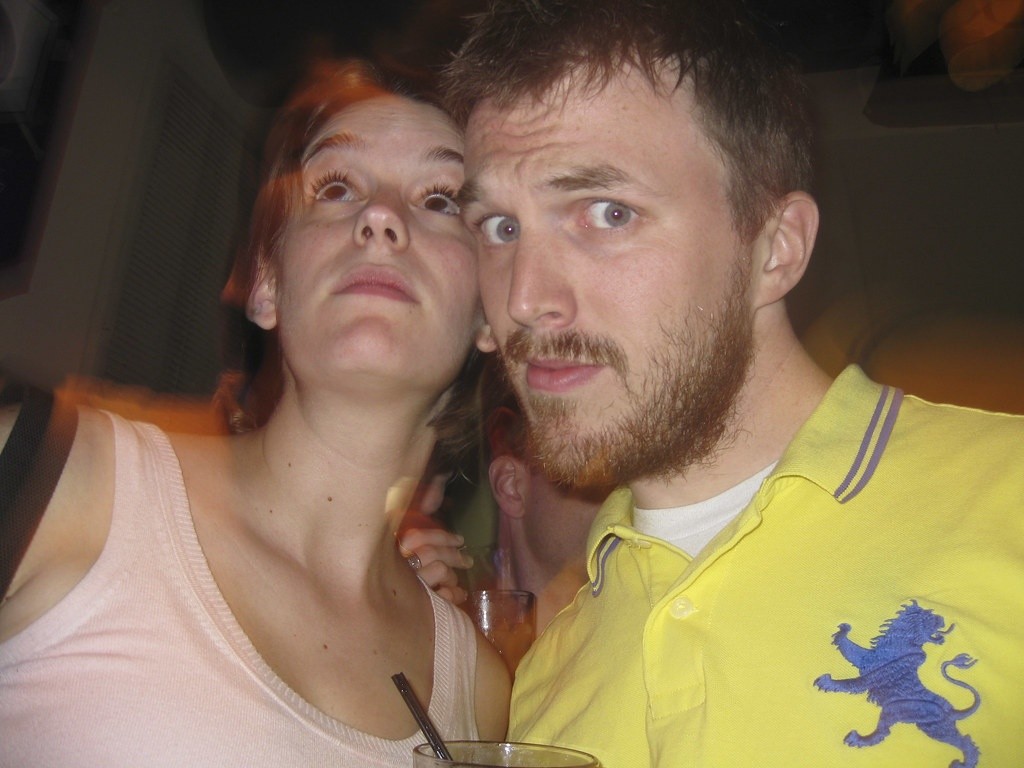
467, 589, 537, 686
412, 740, 601, 768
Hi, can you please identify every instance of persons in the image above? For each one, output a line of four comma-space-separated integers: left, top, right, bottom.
0, 58, 512, 767
434, 1, 1023, 768
